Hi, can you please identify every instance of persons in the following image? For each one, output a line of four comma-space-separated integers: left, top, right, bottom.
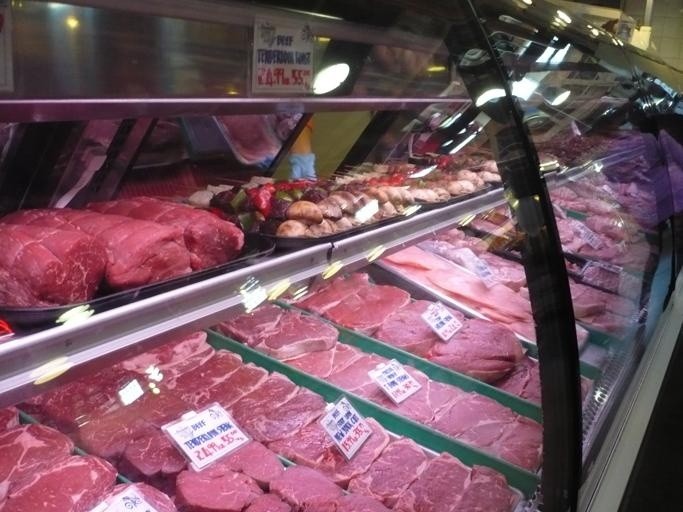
597, 114, 683, 325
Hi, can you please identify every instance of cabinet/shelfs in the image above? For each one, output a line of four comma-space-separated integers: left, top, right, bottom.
1, 0, 682, 512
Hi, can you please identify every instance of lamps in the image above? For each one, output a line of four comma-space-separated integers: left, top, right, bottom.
475, 84, 510, 112
544, 80, 572, 108
309, 54, 354, 99
